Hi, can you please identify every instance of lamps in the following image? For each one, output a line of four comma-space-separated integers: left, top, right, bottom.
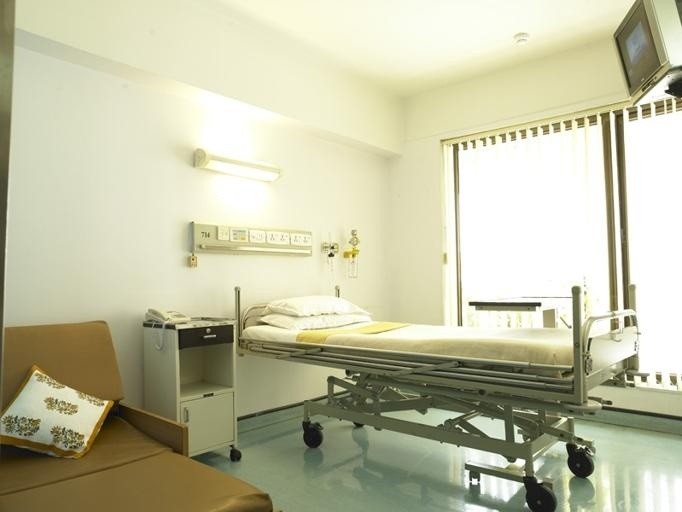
194, 147, 280, 183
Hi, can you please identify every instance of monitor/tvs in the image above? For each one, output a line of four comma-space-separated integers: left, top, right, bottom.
612, 0, 682, 109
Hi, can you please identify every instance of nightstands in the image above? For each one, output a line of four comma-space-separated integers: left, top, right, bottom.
142, 316, 242, 462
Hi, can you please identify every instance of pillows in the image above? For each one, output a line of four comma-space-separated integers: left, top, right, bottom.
258, 313, 372, 330
263, 296, 374, 317
0, 364, 114, 459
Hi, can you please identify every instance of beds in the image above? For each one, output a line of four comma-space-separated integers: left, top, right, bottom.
234, 286, 640, 512
0, 321, 274, 512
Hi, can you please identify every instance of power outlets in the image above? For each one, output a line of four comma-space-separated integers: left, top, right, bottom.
267, 231, 289, 244
249, 230, 266, 243
291, 233, 312, 245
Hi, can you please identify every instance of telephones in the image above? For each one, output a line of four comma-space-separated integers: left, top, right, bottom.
145, 308, 191, 350
